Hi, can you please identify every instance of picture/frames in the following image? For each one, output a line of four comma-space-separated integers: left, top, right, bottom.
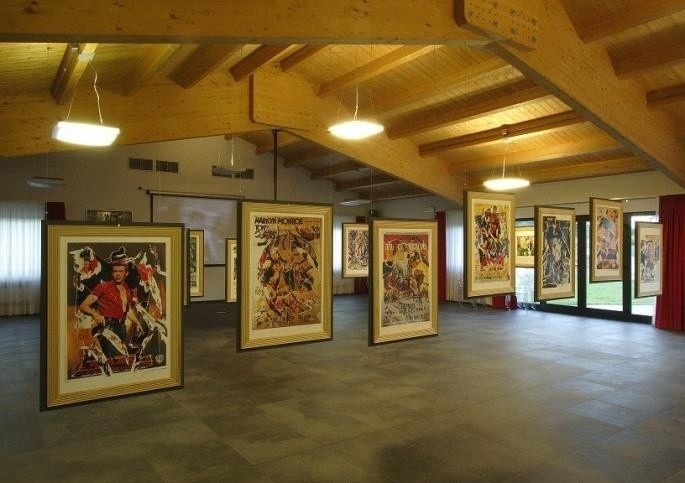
236, 199, 333, 354
589, 196, 624, 283
342, 223, 369, 278
515, 226, 534, 267
534, 204, 576, 302
183, 228, 204, 306
39, 220, 185, 412
85, 209, 133, 221
224, 237, 237, 303
635, 221, 663, 298
368, 217, 439, 346
463, 191, 516, 299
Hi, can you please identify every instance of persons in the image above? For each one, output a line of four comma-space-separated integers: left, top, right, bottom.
80, 260, 144, 360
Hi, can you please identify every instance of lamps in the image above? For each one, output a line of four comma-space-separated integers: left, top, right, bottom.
327, 83, 385, 140
51, 60, 120, 146
483, 143, 530, 191
